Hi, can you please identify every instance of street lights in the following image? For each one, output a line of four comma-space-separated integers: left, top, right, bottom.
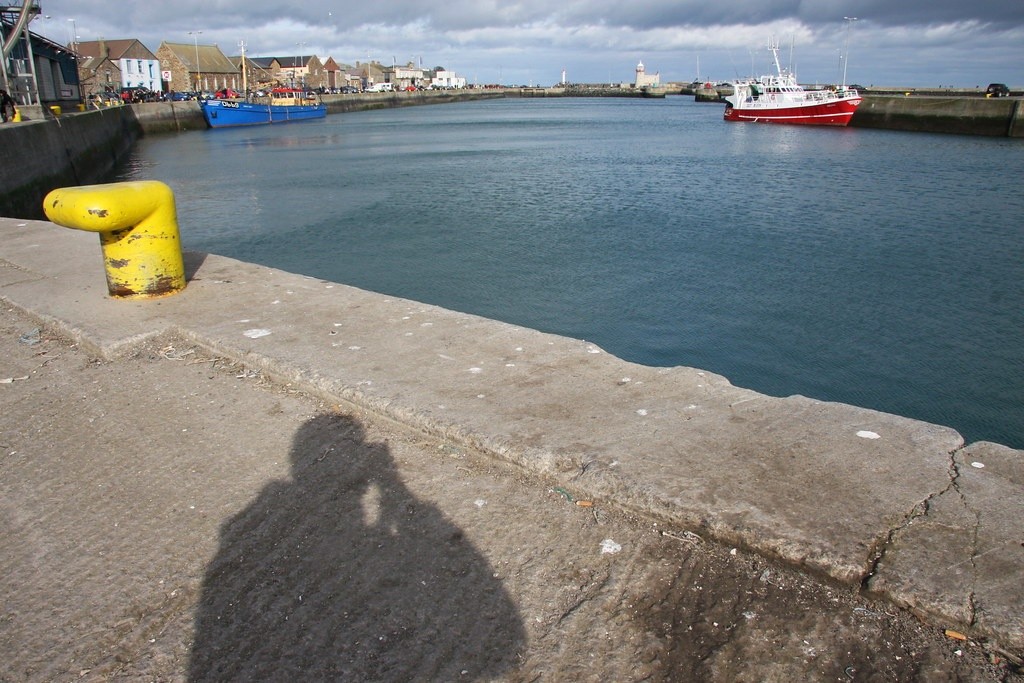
295, 41, 308, 88
842, 16, 858, 86
188, 30, 204, 91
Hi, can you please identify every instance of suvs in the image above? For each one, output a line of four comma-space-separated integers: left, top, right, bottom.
987, 83, 1011, 97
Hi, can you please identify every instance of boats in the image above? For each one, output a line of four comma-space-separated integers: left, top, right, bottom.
723, 30, 863, 127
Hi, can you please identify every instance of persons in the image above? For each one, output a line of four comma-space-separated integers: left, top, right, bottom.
0, 89, 17, 122
90, 85, 400, 107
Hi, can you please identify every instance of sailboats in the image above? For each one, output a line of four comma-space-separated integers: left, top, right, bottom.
198, 38, 328, 129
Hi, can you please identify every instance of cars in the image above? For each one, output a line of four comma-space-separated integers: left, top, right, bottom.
325, 83, 452, 92
165, 92, 217, 102
849, 84, 868, 94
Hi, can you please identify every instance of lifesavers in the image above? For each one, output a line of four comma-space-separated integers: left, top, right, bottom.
771, 94, 775, 100
